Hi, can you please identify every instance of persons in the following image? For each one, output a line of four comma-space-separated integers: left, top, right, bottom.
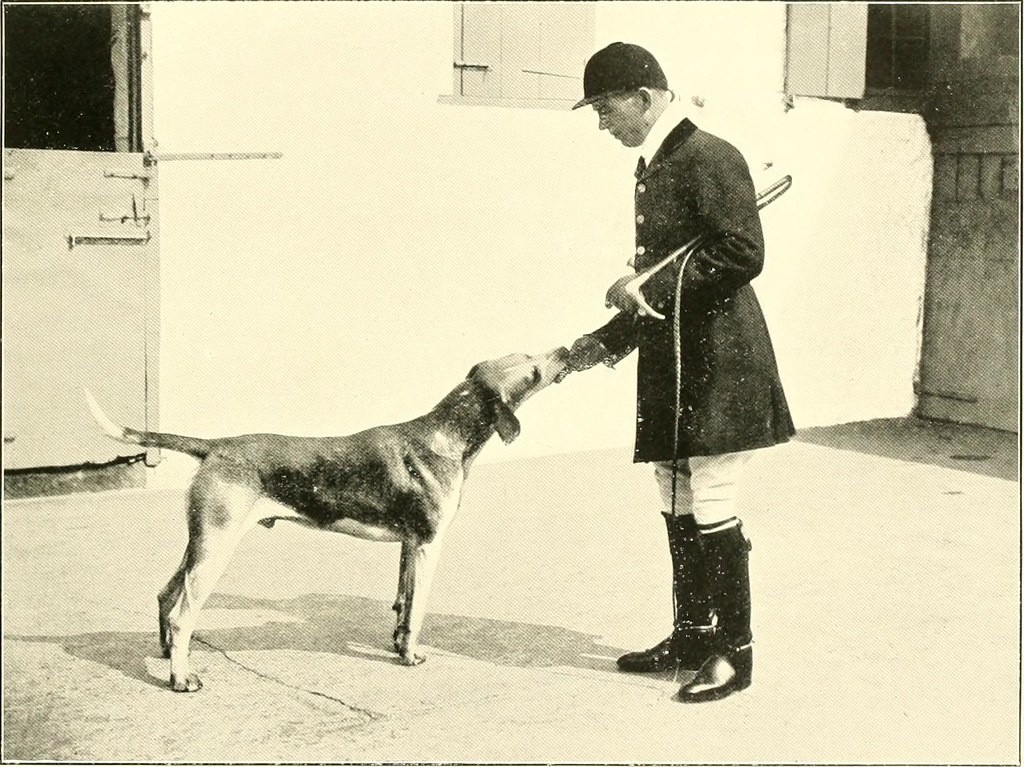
562, 41, 796, 704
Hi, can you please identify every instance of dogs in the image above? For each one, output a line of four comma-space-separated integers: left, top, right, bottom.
86, 345, 572, 695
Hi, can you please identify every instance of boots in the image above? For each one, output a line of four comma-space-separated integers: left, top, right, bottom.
617, 512, 715, 673
679, 517, 752, 703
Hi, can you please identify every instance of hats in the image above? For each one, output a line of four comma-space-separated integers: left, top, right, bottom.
572, 42, 667, 111
